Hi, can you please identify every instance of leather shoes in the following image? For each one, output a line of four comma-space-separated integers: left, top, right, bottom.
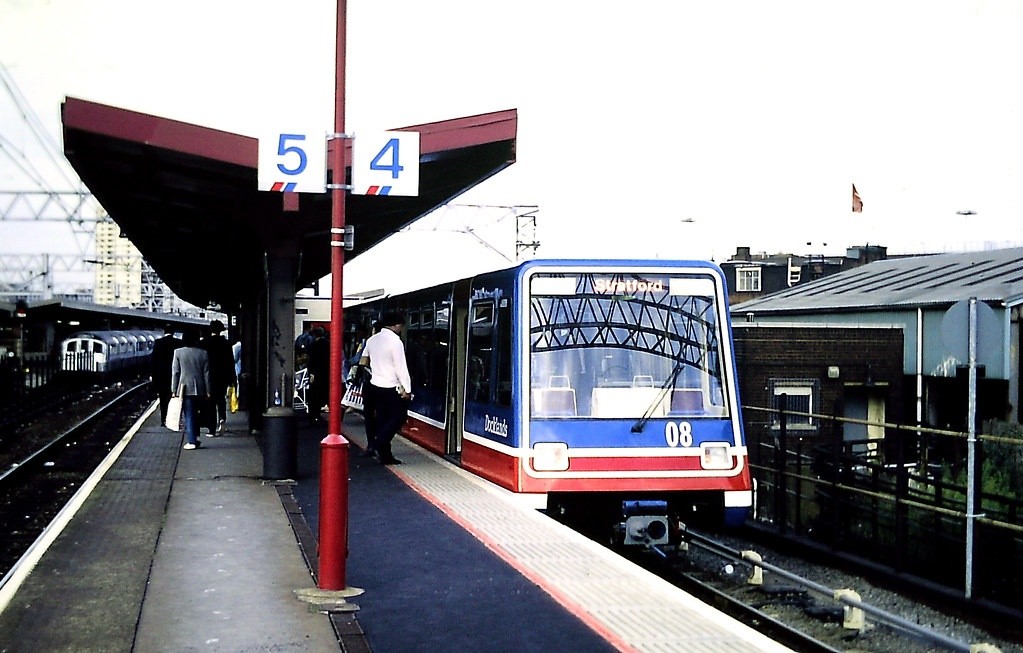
379, 457, 402, 466
359, 448, 373, 457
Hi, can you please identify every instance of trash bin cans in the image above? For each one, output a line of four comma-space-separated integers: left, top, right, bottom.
261, 407, 298, 479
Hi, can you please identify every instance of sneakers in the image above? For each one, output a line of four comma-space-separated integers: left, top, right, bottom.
183, 443, 196, 450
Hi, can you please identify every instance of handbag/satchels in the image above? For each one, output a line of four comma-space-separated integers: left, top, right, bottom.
341, 366, 370, 412
165, 395, 184, 430
227, 386, 239, 414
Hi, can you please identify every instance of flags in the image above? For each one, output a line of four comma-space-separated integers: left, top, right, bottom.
852, 183, 863, 213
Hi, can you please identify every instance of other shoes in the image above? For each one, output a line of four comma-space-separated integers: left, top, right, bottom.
205, 433, 216, 438
216, 420, 225, 436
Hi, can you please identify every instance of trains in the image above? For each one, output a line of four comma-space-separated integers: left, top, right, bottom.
63, 328, 165, 379
332, 256, 755, 551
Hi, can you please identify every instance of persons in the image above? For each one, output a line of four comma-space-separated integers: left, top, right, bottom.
293, 321, 330, 420
149, 324, 181, 427
341, 318, 381, 458
198, 320, 237, 437
232, 333, 243, 401
360, 313, 412, 465
170, 327, 211, 450
